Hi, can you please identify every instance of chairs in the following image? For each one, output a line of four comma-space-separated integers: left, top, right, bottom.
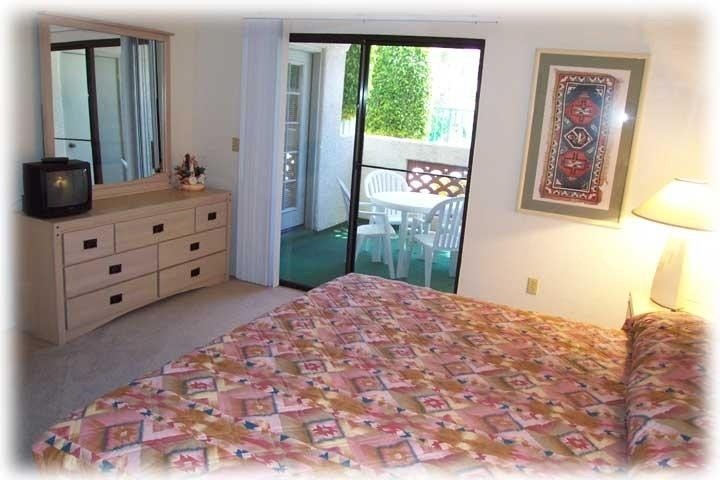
332, 164, 468, 291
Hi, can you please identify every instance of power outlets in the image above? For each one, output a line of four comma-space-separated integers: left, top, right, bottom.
527, 277, 539, 294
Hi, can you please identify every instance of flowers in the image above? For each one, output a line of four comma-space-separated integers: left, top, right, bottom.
173, 152, 207, 191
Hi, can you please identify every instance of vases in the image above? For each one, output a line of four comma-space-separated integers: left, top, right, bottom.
181, 184, 204, 191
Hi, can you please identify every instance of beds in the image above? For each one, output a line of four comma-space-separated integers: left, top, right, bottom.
31, 272, 714, 475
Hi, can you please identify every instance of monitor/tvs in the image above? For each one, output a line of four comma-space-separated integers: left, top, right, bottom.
23, 160, 92, 219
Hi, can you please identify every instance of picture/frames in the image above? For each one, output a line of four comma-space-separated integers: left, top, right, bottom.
515, 47, 652, 229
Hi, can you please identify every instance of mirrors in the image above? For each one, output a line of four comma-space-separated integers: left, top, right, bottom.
50, 25, 167, 188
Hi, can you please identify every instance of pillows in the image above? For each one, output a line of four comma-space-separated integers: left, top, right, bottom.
625, 309, 716, 476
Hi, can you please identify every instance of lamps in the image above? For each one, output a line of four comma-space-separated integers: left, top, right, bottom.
632, 176, 719, 310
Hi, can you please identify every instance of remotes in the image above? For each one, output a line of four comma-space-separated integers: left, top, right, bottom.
41, 157, 69, 161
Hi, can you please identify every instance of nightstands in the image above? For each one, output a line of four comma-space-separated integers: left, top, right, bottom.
625, 291, 671, 318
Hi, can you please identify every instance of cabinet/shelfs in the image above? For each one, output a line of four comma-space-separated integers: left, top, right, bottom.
13, 187, 233, 344
